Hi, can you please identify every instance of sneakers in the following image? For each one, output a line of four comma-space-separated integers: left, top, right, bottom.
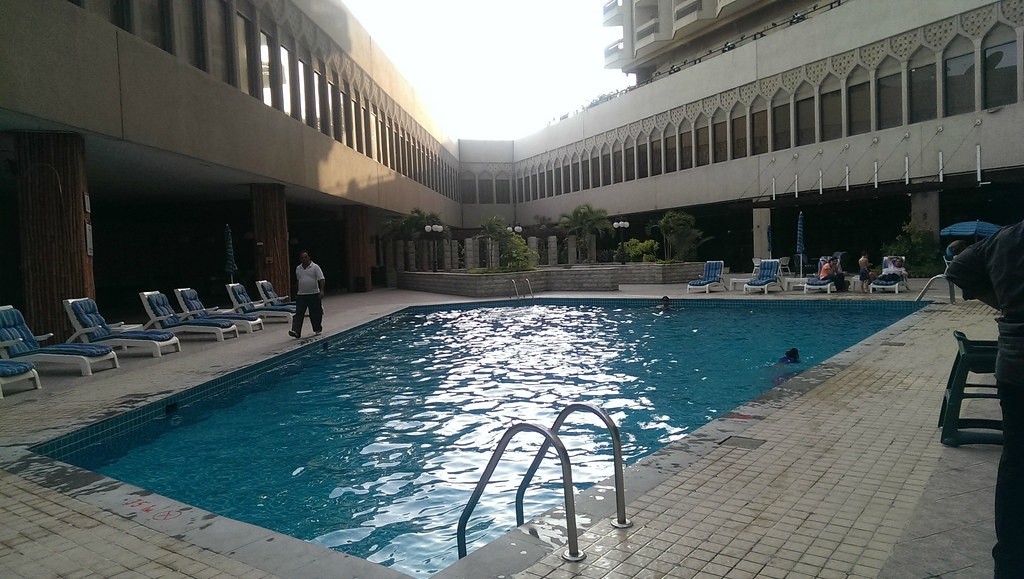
316, 331, 322, 336
288, 330, 300, 339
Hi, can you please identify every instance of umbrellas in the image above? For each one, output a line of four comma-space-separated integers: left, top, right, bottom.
767, 224, 772, 260
223, 224, 238, 284
796, 213, 804, 279
940, 219, 1002, 242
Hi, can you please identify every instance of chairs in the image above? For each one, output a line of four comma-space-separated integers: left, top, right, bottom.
938, 330, 1005, 445
944, 254, 954, 273
0, 280, 324, 400
687, 255, 909, 296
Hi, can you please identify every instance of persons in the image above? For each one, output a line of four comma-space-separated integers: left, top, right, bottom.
947, 240, 967, 260
288, 251, 325, 339
858, 250, 873, 293
779, 348, 800, 363
879, 255, 908, 282
661, 296, 670, 307
947, 218, 1024, 579
819, 257, 847, 293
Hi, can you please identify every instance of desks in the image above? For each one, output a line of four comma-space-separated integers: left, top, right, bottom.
730, 278, 750, 290
761, 259, 779, 263
216, 308, 234, 314
851, 276, 862, 291
111, 324, 144, 331
784, 278, 809, 292
